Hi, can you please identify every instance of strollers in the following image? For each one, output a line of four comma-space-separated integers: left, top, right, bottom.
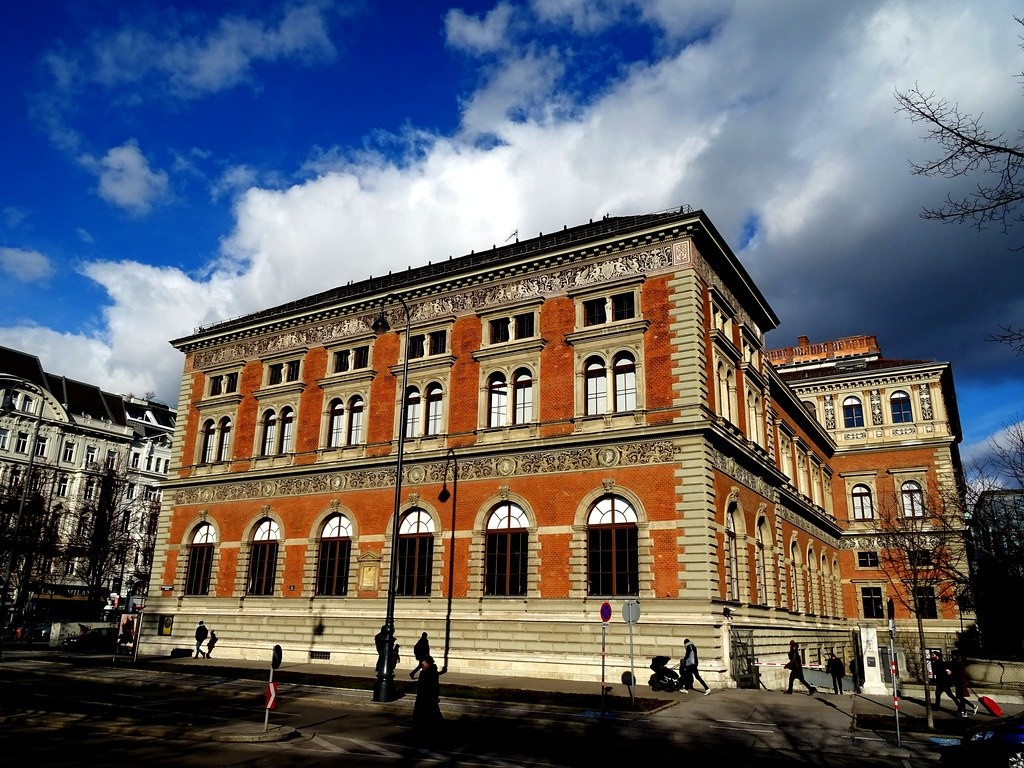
647, 654, 679, 694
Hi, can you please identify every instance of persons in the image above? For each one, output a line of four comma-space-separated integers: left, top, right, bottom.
680, 638, 711, 695
409, 632, 430, 680
784, 640, 818, 695
375, 626, 388, 677
826, 654, 845, 695
194, 621, 208, 659
412, 657, 440, 735
932, 651, 979, 717
850, 654, 863, 691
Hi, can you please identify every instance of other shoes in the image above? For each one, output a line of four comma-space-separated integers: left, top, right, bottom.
194, 655, 198, 659
409, 672, 414, 679
809, 687, 816, 694
202, 652, 205, 659
783, 688, 792, 695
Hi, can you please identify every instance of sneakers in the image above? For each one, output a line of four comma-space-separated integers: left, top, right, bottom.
961, 712, 968, 718
679, 689, 689, 694
704, 688, 711, 695
973, 704, 979, 715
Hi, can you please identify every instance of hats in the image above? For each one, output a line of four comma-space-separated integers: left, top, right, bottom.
199, 621, 203, 625
684, 638, 690, 644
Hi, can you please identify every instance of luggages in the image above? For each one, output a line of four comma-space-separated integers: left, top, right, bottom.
968, 686, 1003, 717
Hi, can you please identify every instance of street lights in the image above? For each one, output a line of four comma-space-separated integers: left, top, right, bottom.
0, 382, 45, 619
371, 292, 414, 707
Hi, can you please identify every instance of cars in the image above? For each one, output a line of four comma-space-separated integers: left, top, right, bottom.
958, 711, 1024, 767
61, 627, 120, 652
